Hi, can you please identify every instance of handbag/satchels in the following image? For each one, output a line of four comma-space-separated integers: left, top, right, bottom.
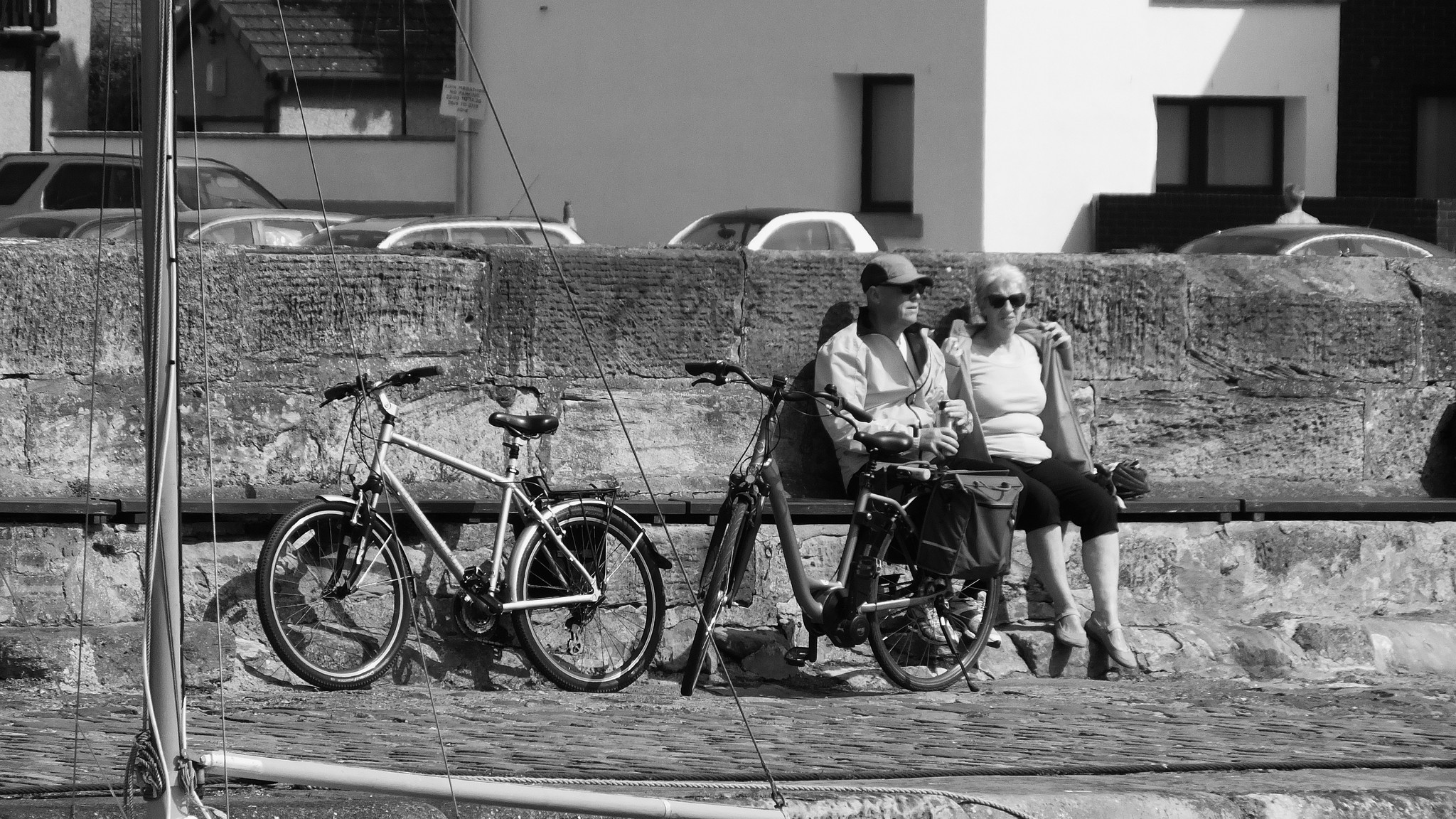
916, 468, 1023, 577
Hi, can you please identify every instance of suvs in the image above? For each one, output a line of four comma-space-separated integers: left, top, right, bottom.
289, 212, 594, 253
91, 208, 367, 247
0, 149, 290, 242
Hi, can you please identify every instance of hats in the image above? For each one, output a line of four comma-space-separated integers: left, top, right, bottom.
862, 254, 934, 290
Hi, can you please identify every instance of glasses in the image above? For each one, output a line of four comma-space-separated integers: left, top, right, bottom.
984, 293, 1026, 307
884, 284, 926, 294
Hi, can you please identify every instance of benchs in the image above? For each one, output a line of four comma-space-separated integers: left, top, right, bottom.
0, 496, 1456, 524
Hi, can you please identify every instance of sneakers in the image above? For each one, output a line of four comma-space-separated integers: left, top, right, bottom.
948, 590, 1002, 649
904, 603, 959, 645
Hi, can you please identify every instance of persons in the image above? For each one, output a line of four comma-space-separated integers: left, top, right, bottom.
938, 263, 1139, 671
815, 252, 1002, 652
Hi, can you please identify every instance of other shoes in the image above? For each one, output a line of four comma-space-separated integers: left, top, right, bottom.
1085, 610, 1139, 669
1055, 611, 1086, 647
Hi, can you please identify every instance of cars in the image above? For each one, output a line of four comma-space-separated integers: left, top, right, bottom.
664, 206, 881, 254
1166, 223, 1456, 263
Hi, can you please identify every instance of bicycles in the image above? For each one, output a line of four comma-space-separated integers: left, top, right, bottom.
253, 366, 675, 694
681, 359, 1022, 700
